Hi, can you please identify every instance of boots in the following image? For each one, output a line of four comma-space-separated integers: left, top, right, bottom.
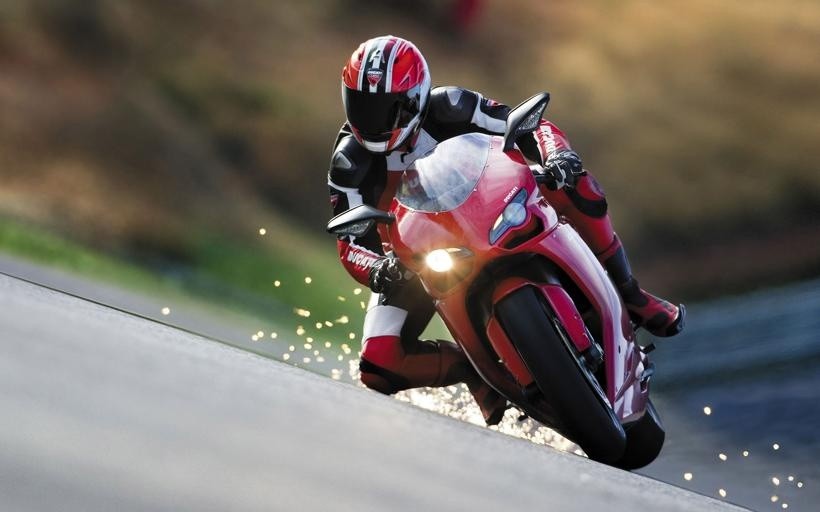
597, 231, 682, 337
424, 339, 505, 425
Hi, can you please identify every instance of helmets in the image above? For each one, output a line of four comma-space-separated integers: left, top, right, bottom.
341, 36, 432, 155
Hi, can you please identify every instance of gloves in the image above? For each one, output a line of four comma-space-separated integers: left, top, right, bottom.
544, 148, 582, 191
370, 257, 401, 293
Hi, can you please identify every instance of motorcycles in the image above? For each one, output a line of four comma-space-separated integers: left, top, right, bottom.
326, 92, 667, 471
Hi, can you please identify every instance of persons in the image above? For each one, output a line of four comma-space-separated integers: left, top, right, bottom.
326, 36, 686, 425
